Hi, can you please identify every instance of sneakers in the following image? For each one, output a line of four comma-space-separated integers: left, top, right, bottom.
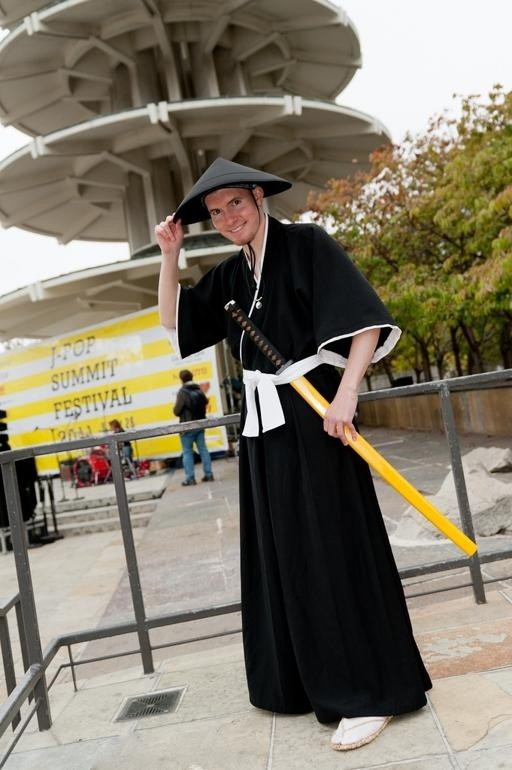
182, 479, 196, 485
202, 476, 214, 481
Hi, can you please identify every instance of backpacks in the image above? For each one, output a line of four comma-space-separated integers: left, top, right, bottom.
180, 388, 209, 419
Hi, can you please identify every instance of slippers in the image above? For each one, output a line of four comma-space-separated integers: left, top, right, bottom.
332, 716, 394, 751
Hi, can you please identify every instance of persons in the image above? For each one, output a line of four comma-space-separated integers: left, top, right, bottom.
109, 419, 132, 457
173, 370, 215, 486
154, 157, 432, 752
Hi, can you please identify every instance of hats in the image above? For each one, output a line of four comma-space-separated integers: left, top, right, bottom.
173, 157, 292, 225
